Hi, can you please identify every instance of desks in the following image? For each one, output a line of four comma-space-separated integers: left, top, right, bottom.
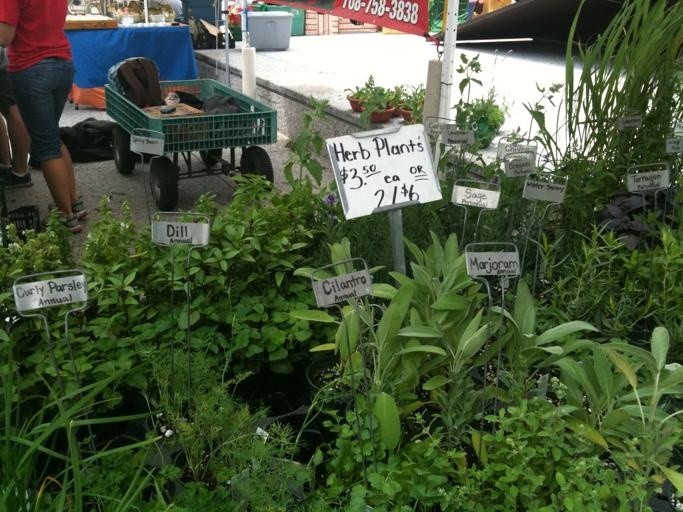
63, 22, 194, 111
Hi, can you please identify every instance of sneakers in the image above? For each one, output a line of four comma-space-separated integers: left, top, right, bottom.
47, 196, 86, 219
40, 213, 81, 233
0, 166, 11, 182
0, 167, 32, 188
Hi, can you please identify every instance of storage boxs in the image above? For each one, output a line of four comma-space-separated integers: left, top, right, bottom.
247, 5, 304, 52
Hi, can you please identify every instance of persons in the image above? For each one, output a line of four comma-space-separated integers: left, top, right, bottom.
1, 1, 89, 234
1, 45, 36, 192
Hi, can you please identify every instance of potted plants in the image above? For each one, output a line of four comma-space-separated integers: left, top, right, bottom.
467, 86, 509, 148
343, 75, 414, 123
289, 235, 375, 448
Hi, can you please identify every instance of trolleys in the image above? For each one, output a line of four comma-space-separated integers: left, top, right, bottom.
104, 74, 278, 214
